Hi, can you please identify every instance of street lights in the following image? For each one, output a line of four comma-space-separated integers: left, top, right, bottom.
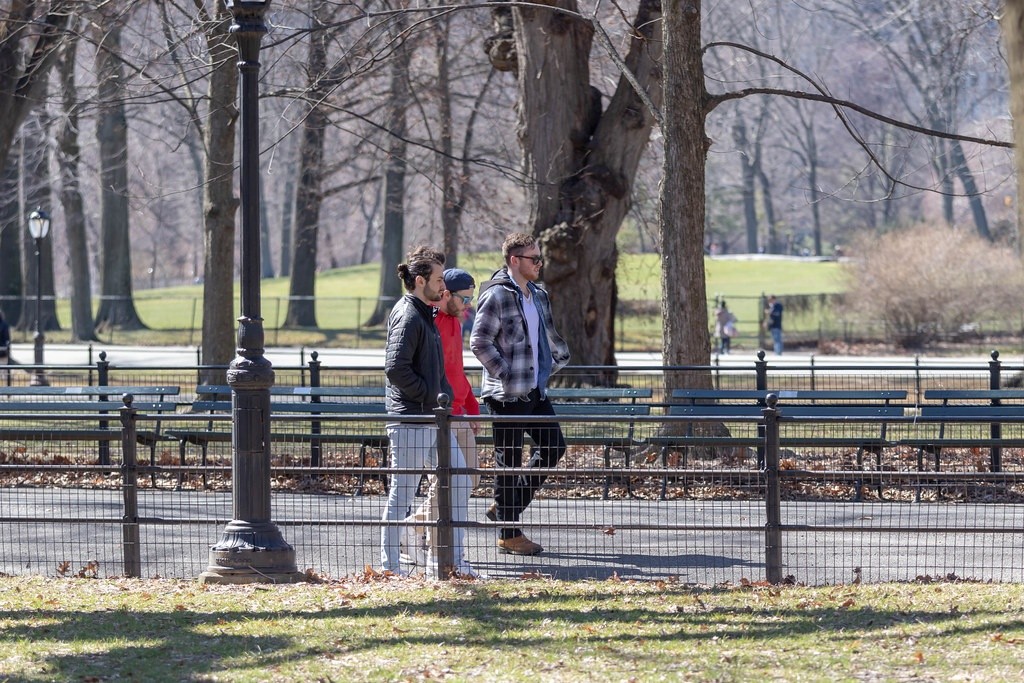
28, 204, 51, 386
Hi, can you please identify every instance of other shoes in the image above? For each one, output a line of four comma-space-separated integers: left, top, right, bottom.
484, 502, 527, 529
382, 568, 410, 577
498, 531, 548, 555
426, 561, 486, 580
398, 516, 429, 565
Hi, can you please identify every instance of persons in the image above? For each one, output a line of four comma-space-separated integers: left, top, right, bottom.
379, 232, 571, 582
712, 294, 786, 355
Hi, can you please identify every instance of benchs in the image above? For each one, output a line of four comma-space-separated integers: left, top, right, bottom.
0, 386, 179, 490
416, 388, 653, 500
900, 390, 1024, 504
645, 389, 908, 500
164, 385, 391, 496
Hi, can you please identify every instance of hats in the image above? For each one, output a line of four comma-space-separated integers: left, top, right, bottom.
441, 268, 476, 290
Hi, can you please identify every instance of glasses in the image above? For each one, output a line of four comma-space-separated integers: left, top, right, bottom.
451, 292, 474, 304
515, 255, 546, 266
